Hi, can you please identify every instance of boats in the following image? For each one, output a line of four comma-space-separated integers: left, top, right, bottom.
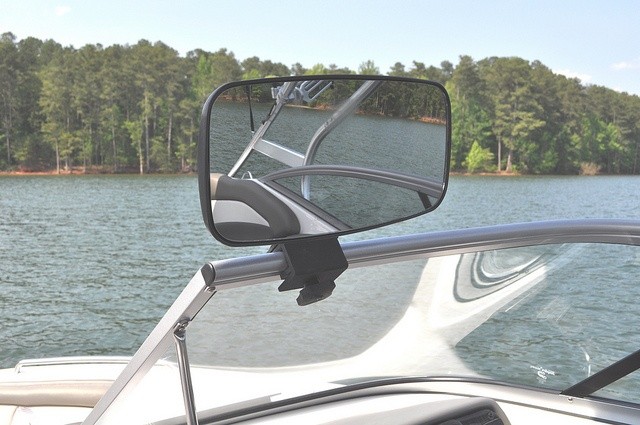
0, 75, 640, 425
